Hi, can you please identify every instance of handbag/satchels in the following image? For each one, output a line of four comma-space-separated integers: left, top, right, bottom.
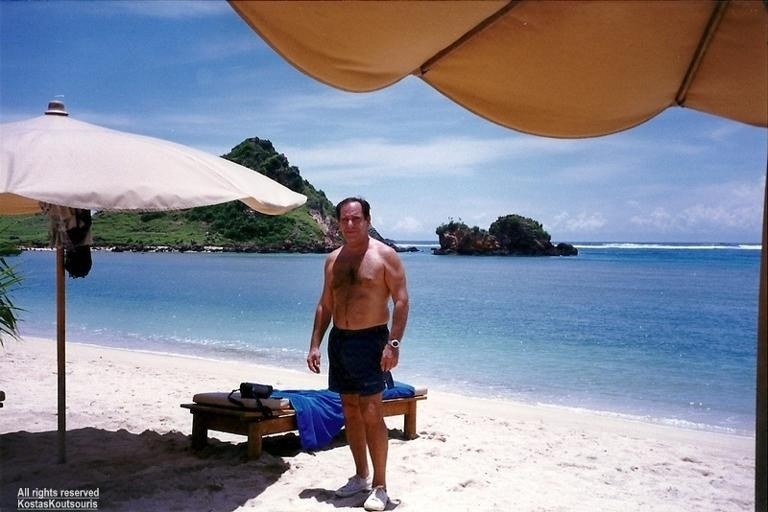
240, 382, 273, 398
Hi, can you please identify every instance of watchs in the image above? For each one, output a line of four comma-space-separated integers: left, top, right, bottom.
387, 339, 400, 348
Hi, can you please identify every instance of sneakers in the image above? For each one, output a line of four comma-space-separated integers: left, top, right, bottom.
363, 484, 389, 511
335, 469, 373, 498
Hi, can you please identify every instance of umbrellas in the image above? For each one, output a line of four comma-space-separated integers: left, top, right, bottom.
0, 102, 308, 464
228, 0, 768, 511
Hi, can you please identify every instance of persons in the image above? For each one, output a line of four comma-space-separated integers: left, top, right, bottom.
307, 197, 409, 511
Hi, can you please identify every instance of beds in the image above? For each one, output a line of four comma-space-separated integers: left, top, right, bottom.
179, 382, 428, 460
